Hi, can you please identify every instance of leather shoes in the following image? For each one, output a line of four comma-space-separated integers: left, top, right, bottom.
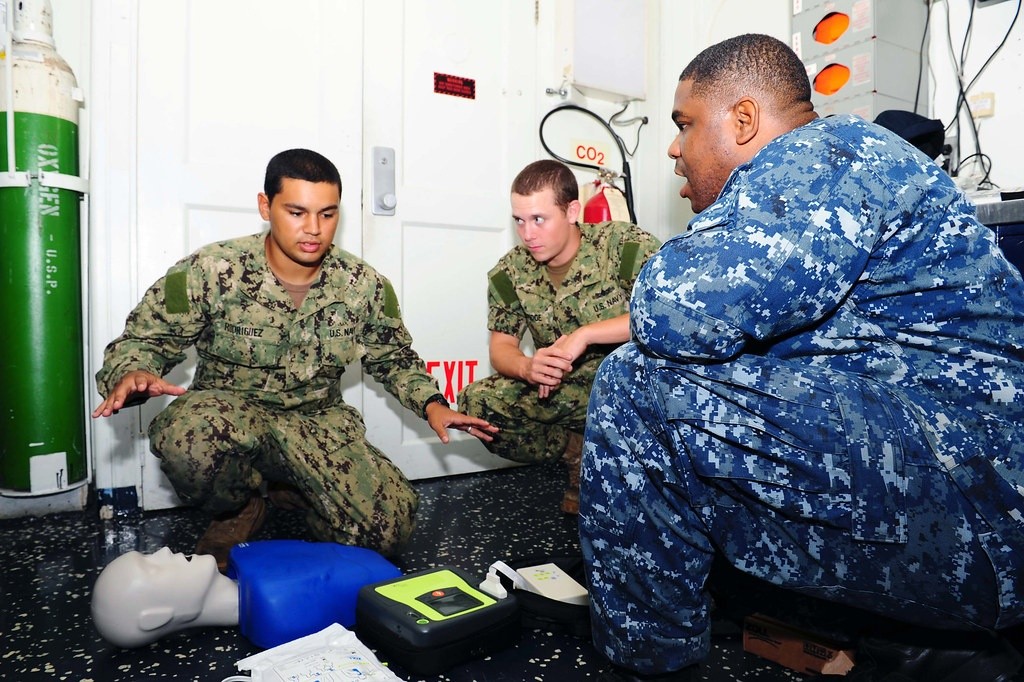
840, 617, 1022, 682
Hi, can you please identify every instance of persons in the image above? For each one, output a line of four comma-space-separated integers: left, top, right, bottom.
583, 31, 1024, 682
92, 159, 499, 570
453, 158, 664, 516
90, 538, 407, 651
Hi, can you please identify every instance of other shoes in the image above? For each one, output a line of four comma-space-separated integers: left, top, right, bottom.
560, 429, 583, 514
195, 484, 269, 570
265, 476, 300, 515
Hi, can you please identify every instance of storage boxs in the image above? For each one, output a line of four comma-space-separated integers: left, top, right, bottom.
742, 623, 854, 676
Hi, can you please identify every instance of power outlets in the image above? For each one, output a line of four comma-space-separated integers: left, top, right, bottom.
939, 136, 958, 176
